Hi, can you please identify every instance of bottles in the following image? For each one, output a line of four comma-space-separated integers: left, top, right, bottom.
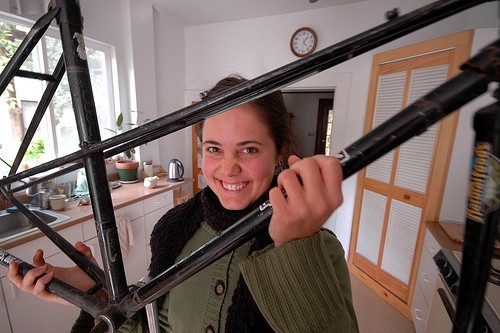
38, 188, 53, 209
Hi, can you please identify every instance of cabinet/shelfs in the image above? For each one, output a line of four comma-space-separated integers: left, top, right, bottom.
410, 229, 445, 333
139, 189, 178, 267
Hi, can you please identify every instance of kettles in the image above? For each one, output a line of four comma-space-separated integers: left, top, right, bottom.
167, 158, 184, 183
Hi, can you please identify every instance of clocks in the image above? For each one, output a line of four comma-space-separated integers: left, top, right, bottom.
290, 27, 317, 58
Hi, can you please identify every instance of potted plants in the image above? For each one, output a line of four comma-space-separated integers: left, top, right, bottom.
107, 116, 147, 183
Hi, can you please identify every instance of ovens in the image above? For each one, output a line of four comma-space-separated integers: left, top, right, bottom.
423, 271, 494, 333
433, 247, 500, 333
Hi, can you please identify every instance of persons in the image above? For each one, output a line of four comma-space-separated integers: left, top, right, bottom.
8, 76, 362, 333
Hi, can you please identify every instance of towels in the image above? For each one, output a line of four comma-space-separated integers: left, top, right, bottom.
115, 214, 135, 248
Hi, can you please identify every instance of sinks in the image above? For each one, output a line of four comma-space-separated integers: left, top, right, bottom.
0, 203, 72, 244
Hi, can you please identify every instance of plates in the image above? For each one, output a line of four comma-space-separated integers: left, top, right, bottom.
109, 181, 121, 188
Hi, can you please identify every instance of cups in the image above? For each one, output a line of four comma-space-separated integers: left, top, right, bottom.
144, 177, 157, 188
59, 183, 71, 197
49, 195, 66, 210
